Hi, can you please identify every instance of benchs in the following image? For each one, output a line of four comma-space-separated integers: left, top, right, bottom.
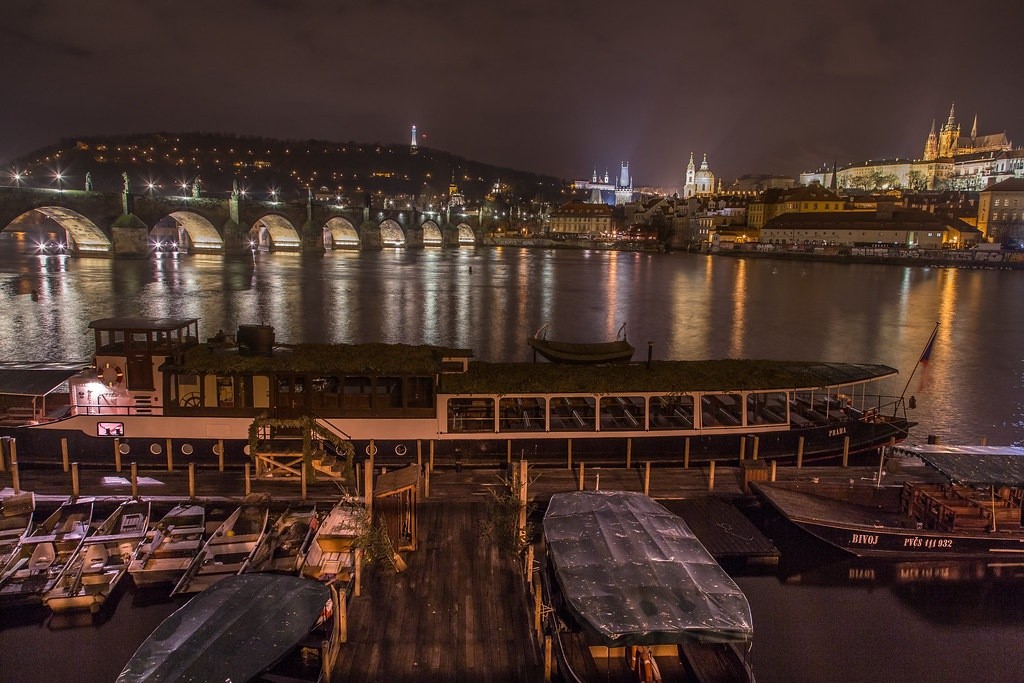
0, 527, 28, 546
899, 478, 1024, 535
0, 405, 40, 427
208, 532, 262, 546
141, 525, 202, 552
194, 562, 244, 576
447, 395, 854, 433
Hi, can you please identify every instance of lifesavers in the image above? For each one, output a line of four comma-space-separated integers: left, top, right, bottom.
97, 363, 124, 389
637, 652, 653, 683
864, 412, 877, 423
624, 644, 637, 672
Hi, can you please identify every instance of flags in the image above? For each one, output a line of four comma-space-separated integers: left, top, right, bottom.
920, 330, 937, 368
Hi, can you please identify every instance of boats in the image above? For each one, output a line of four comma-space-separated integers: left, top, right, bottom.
0, 491, 35, 575
299, 493, 366, 603
541, 473, 758, 683
112, 565, 351, 683
41, 494, 151, 630
747, 440, 1024, 564
0, 316, 940, 473
168, 492, 271, 601
125, 495, 207, 593
244, 497, 319, 576
525, 319, 635, 365
1, 495, 97, 609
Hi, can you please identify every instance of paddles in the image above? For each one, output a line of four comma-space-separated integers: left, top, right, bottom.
0, 556, 29, 584
139, 524, 175, 569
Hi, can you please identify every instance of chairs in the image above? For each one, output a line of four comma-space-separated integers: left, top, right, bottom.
120, 511, 143, 532
54, 512, 85, 534
29, 542, 56, 570
82, 544, 109, 573
